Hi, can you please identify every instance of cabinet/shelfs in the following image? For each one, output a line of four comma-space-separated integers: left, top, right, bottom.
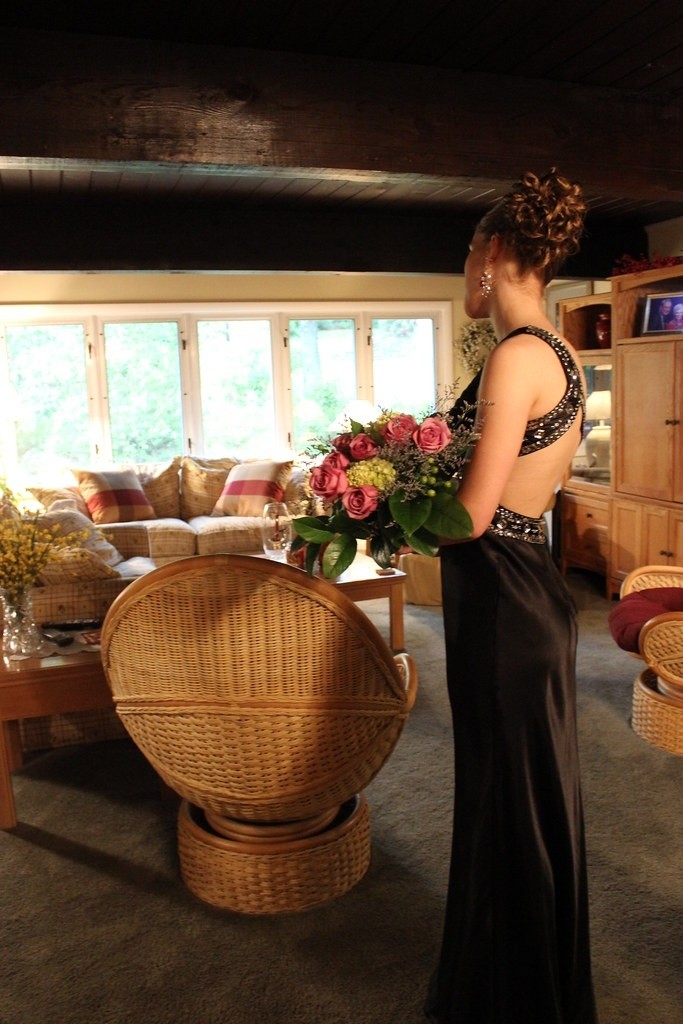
551, 265, 683, 581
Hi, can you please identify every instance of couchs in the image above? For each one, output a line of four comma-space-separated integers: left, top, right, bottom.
0, 477, 159, 626
46, 470, 330, 560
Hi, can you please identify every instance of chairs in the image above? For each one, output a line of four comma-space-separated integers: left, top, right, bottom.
100, 554, 419, 917
607, 565, 683, 758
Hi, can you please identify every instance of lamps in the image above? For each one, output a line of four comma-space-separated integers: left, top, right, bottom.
581, 390, 611, 467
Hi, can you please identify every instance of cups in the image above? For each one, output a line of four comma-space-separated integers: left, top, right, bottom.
262, 502, 293, 565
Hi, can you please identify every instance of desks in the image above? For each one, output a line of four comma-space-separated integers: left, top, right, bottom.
0, 549, 409, 830
609, 585, 683, 655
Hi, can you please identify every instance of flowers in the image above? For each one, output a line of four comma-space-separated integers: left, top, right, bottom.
0, 507, 114, 636
291, 376, 495, 580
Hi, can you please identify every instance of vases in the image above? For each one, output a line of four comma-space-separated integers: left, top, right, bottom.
0, 586, 45, 661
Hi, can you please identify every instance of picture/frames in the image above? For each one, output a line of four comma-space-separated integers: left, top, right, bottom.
641, 292, 683, 336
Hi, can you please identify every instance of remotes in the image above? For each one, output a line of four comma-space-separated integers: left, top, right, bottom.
43, 626, 74, 647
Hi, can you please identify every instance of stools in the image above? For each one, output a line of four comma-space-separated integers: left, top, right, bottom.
398, 551, 442, 606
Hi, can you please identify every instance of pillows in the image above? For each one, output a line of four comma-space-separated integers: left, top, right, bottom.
17, 455, 326, 581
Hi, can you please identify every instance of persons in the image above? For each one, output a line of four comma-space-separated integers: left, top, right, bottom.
369, 168, 600, 1024
646, 299, 683, 330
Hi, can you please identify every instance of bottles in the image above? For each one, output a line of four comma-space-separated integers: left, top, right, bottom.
596, 313, 610, 349
